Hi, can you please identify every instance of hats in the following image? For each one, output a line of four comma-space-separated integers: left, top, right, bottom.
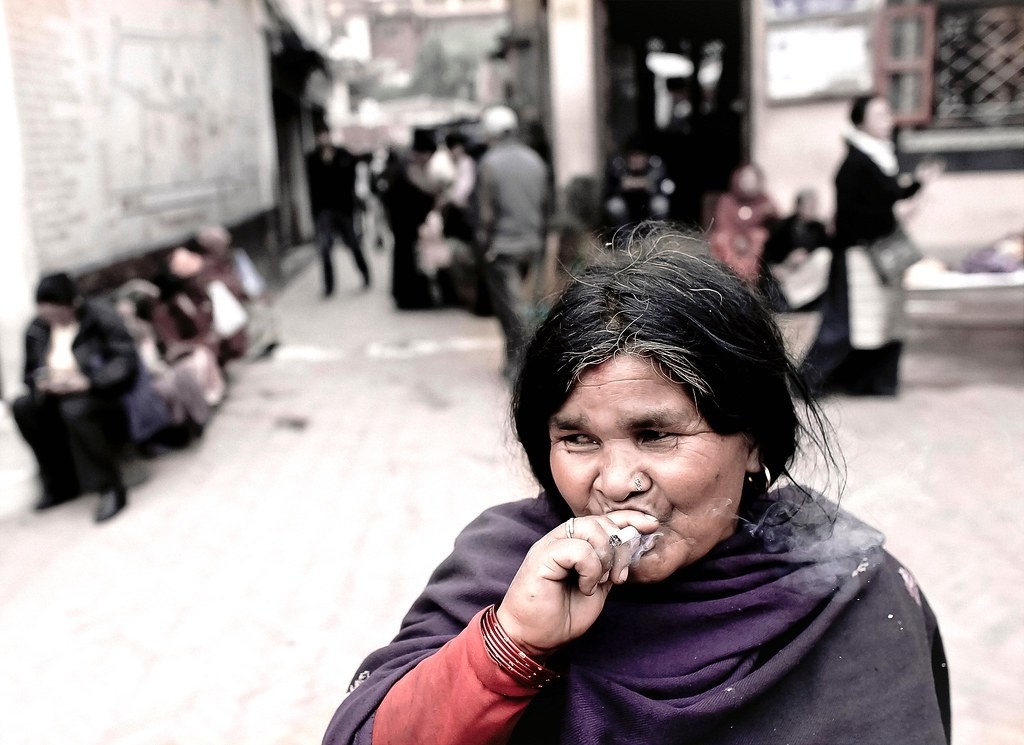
481, 106, 519, 139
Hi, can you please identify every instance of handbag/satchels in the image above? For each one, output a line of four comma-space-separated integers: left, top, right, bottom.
868, 228, 920, 283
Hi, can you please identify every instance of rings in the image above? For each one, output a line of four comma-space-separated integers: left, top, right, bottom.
565, 517, 574, 538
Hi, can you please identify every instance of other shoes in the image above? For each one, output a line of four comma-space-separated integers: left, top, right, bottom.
34, 487, 81, 511
94, 484, 122, 524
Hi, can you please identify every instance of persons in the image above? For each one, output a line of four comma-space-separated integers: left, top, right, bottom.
792, 94, 923, 396
479, 101, 548, 383
12, 221, 286, 523
301, 30, 922, 319
323, 234, 951, 745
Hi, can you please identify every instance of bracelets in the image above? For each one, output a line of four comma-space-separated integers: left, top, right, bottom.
481, 604, 561, 689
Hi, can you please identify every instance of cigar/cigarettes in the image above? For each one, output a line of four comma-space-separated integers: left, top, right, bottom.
608, 525, 639, 550
